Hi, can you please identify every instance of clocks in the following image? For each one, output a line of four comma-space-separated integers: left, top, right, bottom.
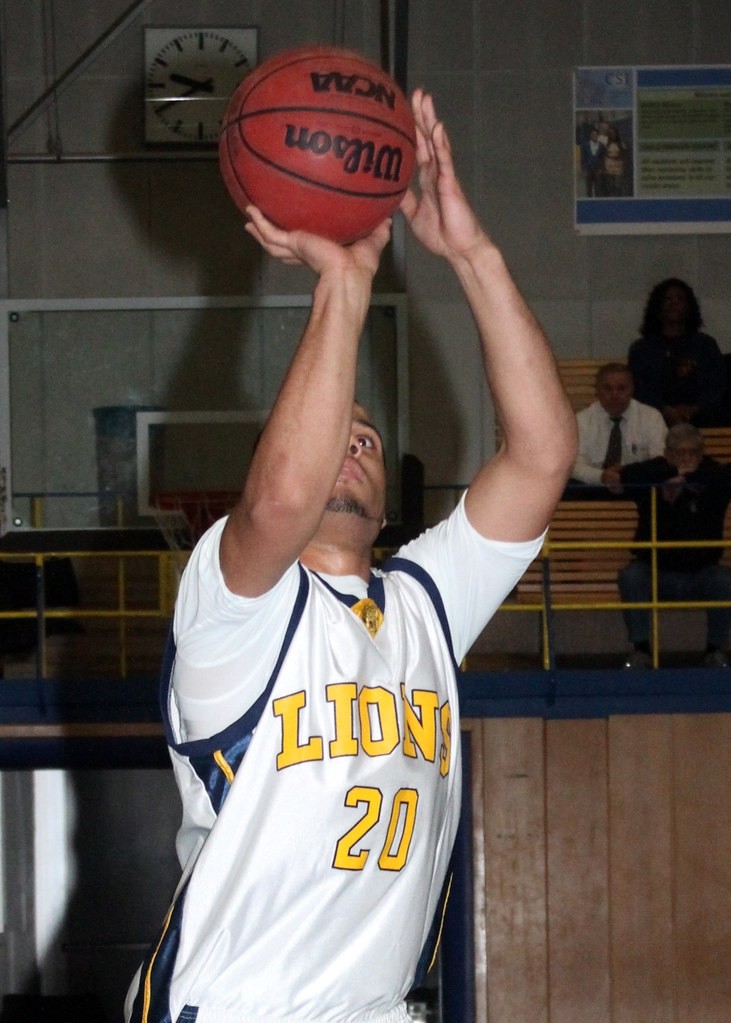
143, 26, 260, 146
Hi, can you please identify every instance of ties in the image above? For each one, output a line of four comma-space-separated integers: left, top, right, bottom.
602, 416, 623, 470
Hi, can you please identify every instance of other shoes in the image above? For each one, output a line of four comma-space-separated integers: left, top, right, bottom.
703, 650, 729, 669
624, 653, 654, 669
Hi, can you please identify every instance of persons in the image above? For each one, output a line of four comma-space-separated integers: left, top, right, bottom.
629, 278, 724, 425
563, 362, 669, 499
123, 88, 581, 1023
582, 119, 627, 197
620, 424, 731, 670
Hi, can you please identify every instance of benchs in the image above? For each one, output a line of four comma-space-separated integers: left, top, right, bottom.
494, 358, 731, 667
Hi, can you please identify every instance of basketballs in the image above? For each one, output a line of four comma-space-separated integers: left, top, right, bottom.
217, 44, 420, 246
